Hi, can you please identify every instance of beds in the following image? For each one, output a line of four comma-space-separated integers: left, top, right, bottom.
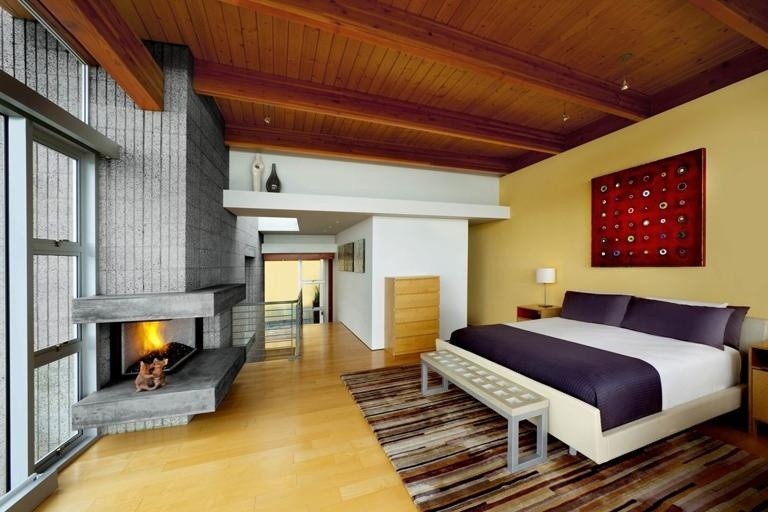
435, 315, 768, 466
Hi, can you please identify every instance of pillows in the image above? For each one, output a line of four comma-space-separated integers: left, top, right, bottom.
559, 290, 750, 350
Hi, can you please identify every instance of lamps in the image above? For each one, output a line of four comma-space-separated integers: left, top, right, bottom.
535, 267, 557, 308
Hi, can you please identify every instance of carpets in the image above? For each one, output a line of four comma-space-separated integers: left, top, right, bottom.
341, 362, 767, 512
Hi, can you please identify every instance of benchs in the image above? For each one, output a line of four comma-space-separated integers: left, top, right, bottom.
420, 349, 550, 474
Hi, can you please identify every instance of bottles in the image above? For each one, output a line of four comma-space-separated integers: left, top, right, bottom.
264, 162, 282, 192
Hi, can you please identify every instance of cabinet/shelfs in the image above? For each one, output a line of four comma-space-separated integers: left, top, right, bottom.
383, 273, 440, 357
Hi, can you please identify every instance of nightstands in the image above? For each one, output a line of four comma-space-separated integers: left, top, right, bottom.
746, 340, 768, 434
515, 304, 563, 323
